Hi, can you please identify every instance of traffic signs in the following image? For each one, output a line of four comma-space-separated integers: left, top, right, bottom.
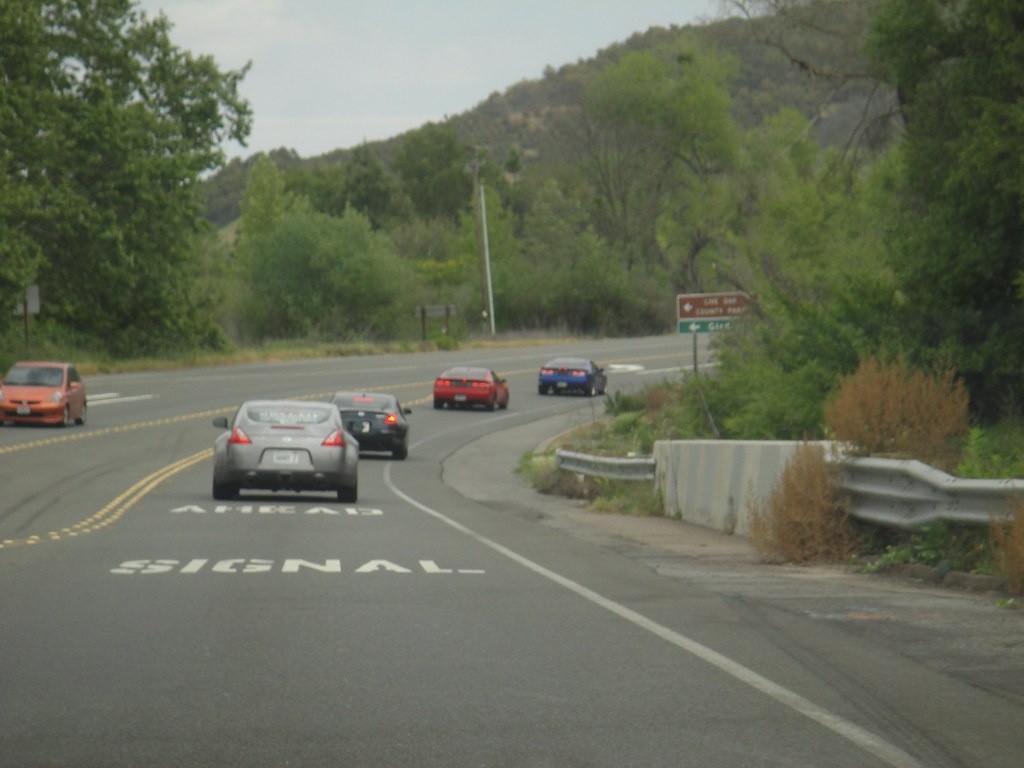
677, 291, 754, 333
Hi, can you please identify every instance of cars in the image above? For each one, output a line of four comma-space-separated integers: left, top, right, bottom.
433, 367, 509, 411
329, 391, 412, 460
0, 361, 88, 427
538, 359, 607, 397
212, 400, 360, 503
